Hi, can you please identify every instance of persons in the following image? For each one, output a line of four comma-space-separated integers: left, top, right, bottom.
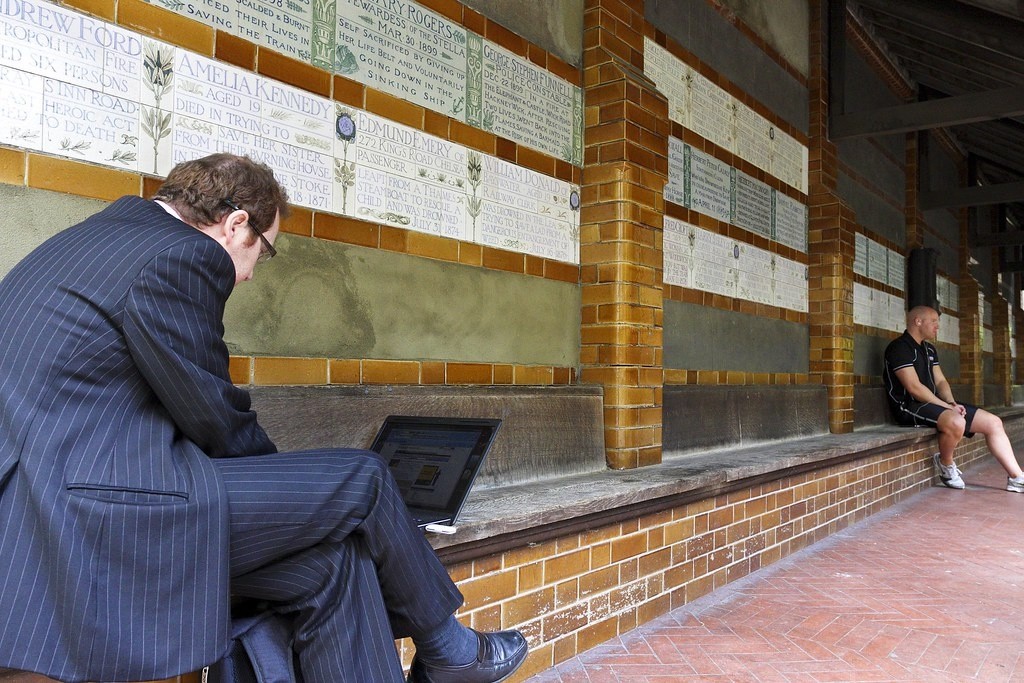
883, 306, 1024, 493
0, 154, 528, 683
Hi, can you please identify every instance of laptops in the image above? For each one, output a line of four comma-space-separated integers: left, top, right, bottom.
368, 415, 503, 533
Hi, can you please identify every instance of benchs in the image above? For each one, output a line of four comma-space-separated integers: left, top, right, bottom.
233, 385, 1024, 683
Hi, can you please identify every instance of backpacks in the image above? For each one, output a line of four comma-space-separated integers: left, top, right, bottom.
201, 610, 303, 683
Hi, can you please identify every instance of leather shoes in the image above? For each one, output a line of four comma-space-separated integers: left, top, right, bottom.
405, 626, 529, 683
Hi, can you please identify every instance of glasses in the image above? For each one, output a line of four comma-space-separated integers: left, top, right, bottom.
222, 197, 278, 264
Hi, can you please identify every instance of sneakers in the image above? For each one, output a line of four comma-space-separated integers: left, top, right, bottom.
1005, 472, 1024, 494
933, 452, 966, 489
